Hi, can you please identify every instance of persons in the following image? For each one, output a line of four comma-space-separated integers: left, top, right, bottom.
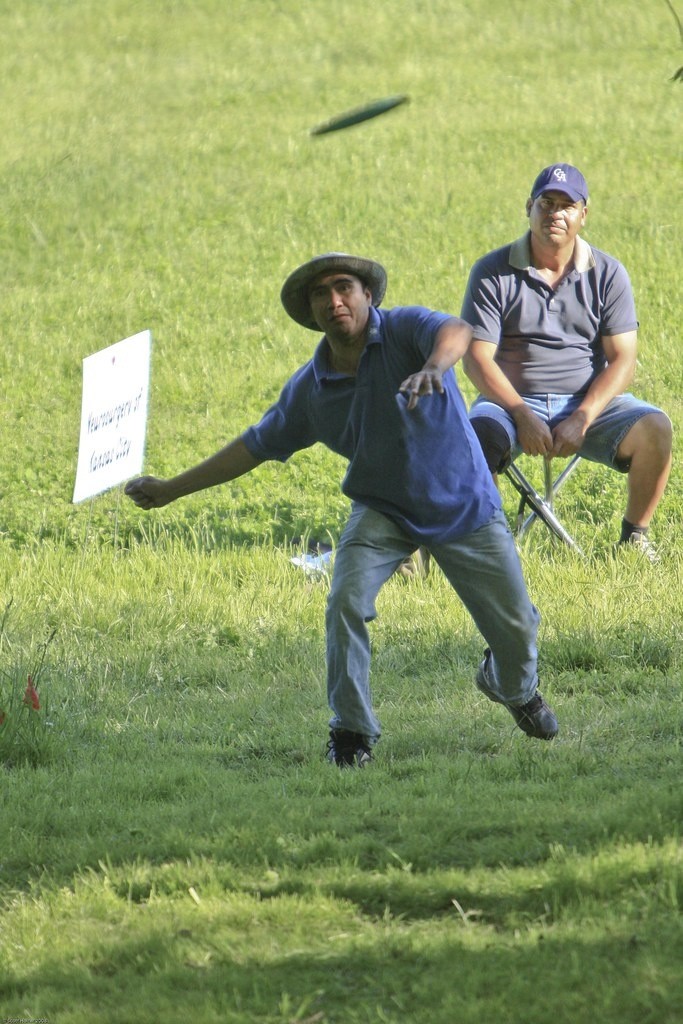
459, 164, 673, 565
121, 250, 560, 767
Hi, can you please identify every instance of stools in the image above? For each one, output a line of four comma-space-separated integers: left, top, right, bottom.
500, 451, 589, 561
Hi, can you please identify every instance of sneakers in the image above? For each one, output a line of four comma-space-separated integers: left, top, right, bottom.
476, 659, 558, 739
614, 531, 661, 563
325, 729, 375, 768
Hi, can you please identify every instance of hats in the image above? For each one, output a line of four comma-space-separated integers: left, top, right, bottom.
532, 163, 589, 206
280, 251, 387, 332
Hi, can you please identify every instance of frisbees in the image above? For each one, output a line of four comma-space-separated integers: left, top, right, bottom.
313, 97, 408, 136
